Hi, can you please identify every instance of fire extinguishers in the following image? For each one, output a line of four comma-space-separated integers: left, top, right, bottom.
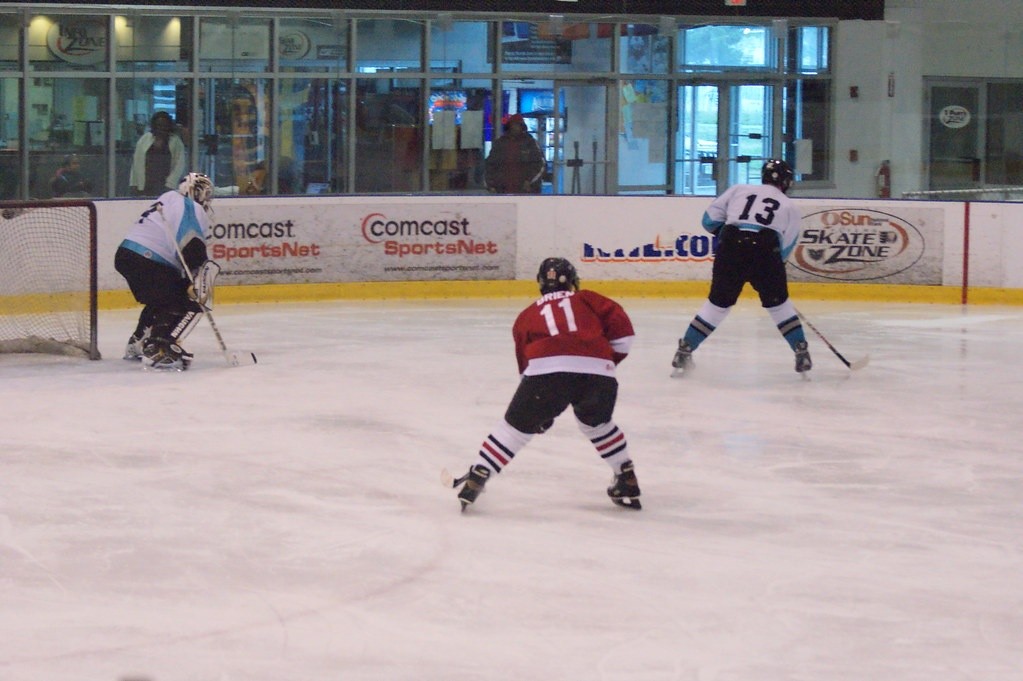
876, 159, 889, 198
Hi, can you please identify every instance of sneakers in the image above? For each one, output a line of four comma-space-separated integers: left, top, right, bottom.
607, 460, 641, 509
124, 333, 144, 361
671, 339, 695, 377
794, 342, 813, 381
451, 465, 491, 513
141, 336, 193, 371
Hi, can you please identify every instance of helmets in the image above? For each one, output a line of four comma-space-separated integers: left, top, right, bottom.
536, 258, 580, 295
181, 172, 214, 212
762, 159, 794, 194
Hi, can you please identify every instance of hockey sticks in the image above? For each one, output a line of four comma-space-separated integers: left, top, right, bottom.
791, 304, 870, 372
155, 203, 259, 367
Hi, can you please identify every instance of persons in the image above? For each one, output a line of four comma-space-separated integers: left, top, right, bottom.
453, 258, 642, 512
54, 155, 91, 195
128, 112, 186, 197
484, 113, 547, 194
670, 158, 812, 380
115, 172, 215, 373
